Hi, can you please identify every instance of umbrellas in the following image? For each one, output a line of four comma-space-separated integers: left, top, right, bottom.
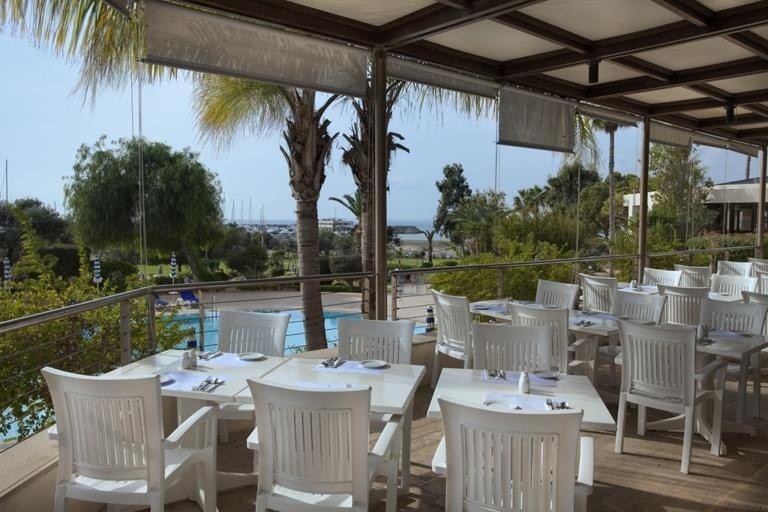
425, 306, 436, 333
168, 250, 180, 294
92, 255, 104, 290
186, 326, 198, 350
3, 256, 13, 294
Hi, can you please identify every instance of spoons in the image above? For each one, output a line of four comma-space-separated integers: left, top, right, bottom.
559, 401, 569, 408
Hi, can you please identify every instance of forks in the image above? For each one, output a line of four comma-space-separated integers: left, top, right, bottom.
320, 356, 337, 366
546, 397, 555, 411
488, 367, 505, 378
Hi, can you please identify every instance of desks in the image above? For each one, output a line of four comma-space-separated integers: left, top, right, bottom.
428, 365, 616, 431
469, 298, 655, 385
236, 347, 427, 501
98, 348, 290, 511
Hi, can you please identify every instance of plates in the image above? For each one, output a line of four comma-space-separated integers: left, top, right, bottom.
531, 369, 560, 378
160, 373, 173, 384
480, 398, 518, 412
609, 313, 626, 318
236, 351, 264, 360
540, 304, 558, 308
471, 303, 488, 309
728, 330, 751, 334
358, 360, 388, 368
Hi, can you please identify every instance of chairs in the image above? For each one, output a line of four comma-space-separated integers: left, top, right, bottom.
534, 261, 767, 475
246, 377, 405, 512
336, 316, 416, 432
470, 322, 552, 373
42, 364, 219, 511
430, 287, 475, 386
431, 393, 596, 511
506, 301, 598, 384
210, 309, 292, 421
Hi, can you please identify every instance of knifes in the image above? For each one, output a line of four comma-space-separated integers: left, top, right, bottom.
696, 338, 715, 345
198, 350, 222, 360
576, 320, 591, 327
196, 376, 224, 392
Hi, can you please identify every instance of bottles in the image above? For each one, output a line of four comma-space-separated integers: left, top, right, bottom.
630, 280, 637, 289
181, 348, 198, 369
697, 322, 709, 338
504, 297, 513, 308
519, 371, 530, 394
